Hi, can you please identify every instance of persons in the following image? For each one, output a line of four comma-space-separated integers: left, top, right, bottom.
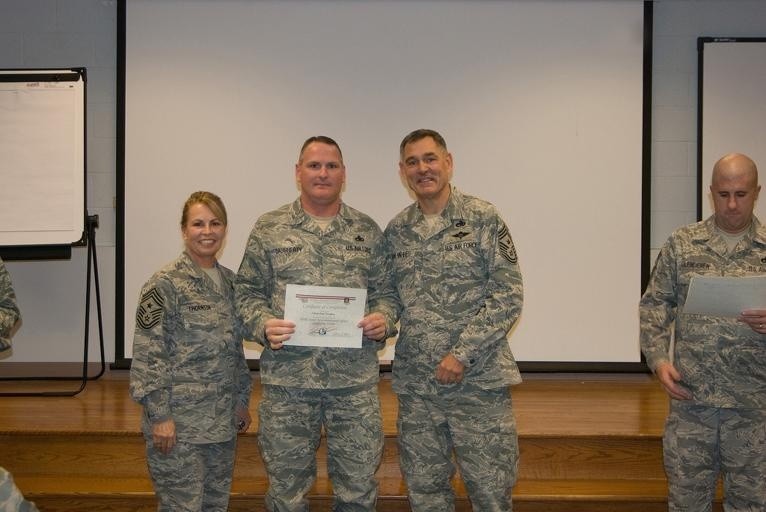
385, 130, 524, 511
639, 153, 766, 512
130, 191, 252, 511
0, 257, 22, 355
1, 461, 37, 512
233, 135, 398, 512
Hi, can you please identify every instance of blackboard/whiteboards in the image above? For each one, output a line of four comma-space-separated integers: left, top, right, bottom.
0, 68, 87, 247
